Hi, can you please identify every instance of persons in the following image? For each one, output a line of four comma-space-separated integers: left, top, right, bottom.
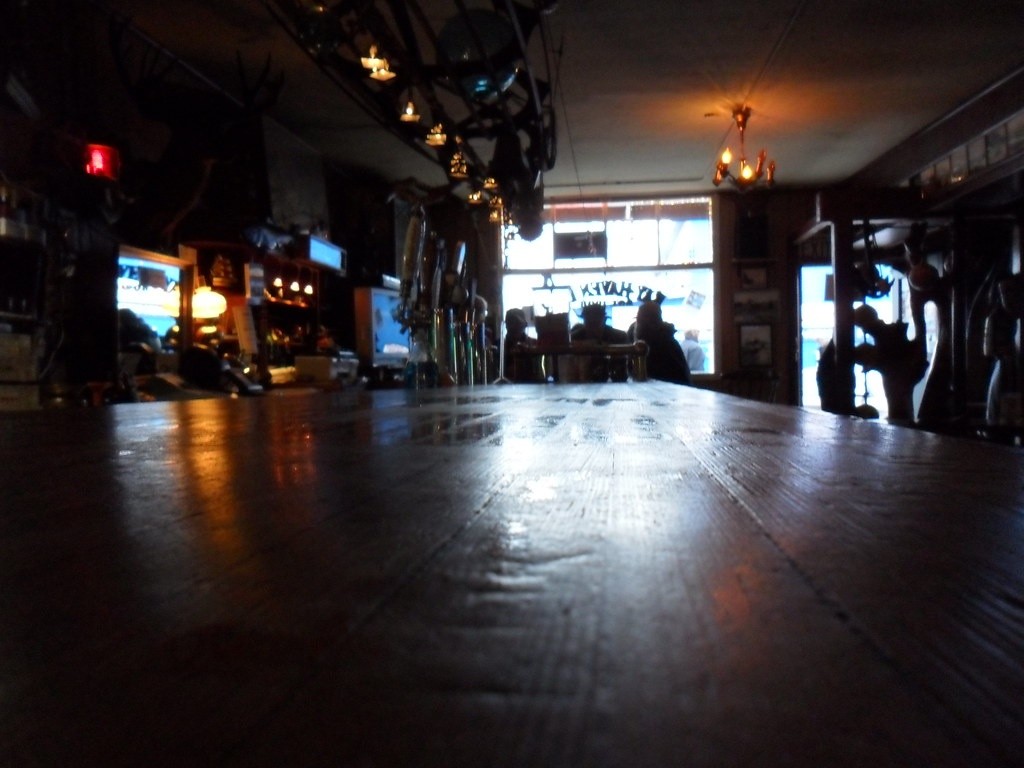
983, 274, 1024, 450
853, 304, 915, 423
118, 310, 161, 402
410, 295, 705, 387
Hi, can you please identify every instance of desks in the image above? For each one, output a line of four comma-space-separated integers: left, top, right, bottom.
544, 339, 649, 383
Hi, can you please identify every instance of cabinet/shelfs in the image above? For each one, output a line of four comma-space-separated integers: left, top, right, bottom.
0, 219, 52, 343
255, 252, 323, 369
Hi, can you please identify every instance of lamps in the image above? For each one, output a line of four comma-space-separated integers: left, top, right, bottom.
711, 109, 778, 195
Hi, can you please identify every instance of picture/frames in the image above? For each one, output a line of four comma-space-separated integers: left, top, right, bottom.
739, 266, 772, 311
739, 322, 774, 369
